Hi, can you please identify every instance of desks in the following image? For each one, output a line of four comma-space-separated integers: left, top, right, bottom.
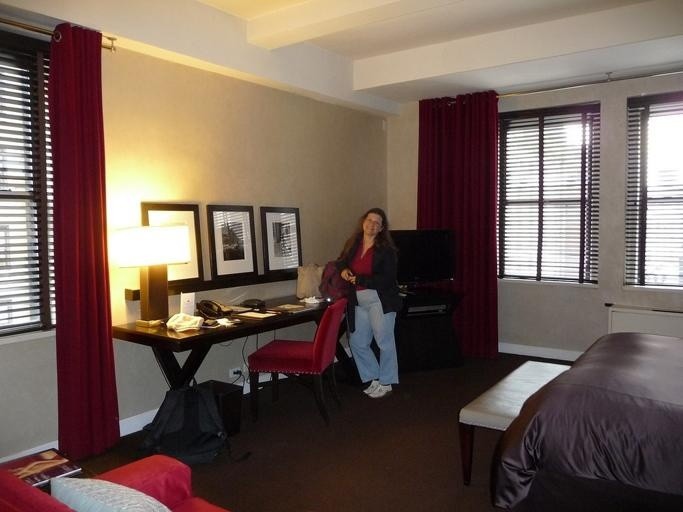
1, 447, 90, 492
109, 293, 355, 455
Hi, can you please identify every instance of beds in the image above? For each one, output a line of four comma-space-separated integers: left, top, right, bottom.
491, 325, 683, 511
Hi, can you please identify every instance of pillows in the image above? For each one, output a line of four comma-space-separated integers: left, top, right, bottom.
47, 475, 173, 512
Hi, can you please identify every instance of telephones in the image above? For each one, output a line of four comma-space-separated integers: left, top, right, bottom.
197, 300, 233, 315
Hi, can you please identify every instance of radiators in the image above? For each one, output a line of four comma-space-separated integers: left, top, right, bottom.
602, 304, 682, 337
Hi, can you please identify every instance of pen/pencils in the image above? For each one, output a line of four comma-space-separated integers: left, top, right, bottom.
266, 310, 282, 314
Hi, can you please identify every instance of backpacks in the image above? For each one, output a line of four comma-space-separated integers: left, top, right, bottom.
139, 376, 230, 463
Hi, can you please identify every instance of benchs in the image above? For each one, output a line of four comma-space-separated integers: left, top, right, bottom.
454, 356, 573, 482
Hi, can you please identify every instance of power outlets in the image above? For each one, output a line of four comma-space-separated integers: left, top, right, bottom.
228, 366, 241, 379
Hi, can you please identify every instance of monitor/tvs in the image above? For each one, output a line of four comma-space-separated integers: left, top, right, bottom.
390, 228, 456, 287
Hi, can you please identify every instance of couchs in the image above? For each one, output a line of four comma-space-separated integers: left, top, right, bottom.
0, 447, 233, 511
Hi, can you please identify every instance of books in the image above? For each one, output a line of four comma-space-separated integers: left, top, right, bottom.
4, 450, 82, 487
224, 302, 305, 318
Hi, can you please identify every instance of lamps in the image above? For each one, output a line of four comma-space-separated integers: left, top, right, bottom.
106, 223, 192, 327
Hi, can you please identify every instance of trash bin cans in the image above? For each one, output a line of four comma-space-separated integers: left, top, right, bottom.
196, 380, 243, 435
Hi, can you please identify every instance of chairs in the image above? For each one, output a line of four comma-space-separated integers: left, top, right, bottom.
249, 296, 349, 428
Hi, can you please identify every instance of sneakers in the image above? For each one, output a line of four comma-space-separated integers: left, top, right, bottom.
363, 380, 392, 399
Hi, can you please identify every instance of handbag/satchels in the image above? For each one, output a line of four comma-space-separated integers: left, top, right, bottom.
296, 264, 325, 299
319, 260, 350, 299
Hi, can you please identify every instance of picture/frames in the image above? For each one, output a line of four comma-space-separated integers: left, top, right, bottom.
138, 197, 203, 288
205, 201, 259, 280
256, 205, 303, 276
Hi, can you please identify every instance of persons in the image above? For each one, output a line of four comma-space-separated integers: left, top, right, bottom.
333, 208, 402, 398
